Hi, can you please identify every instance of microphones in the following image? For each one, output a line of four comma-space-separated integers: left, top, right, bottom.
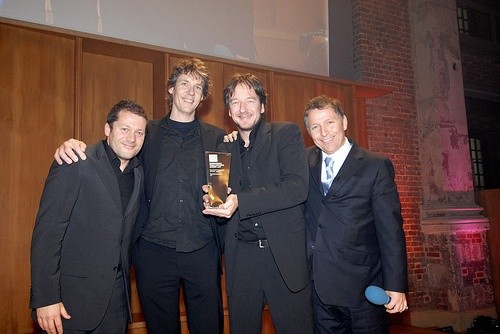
365, 286, 408, 311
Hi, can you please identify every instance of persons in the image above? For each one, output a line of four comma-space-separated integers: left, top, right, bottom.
30, 58, 408, 334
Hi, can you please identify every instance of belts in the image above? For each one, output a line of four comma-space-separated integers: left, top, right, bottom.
248, 239, 269, 248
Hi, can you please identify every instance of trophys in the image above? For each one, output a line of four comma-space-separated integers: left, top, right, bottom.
205, 151, 232, 210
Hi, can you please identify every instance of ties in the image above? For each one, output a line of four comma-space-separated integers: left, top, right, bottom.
321, 157, 335, 196
